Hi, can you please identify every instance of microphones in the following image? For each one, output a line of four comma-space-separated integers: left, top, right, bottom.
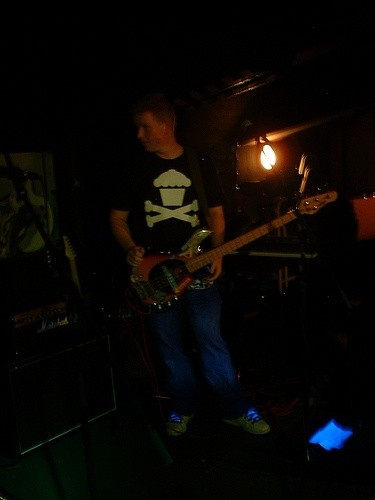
241, 120, 270, 143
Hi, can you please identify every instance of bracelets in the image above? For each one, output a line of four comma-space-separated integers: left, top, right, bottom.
127, 244, 138, 252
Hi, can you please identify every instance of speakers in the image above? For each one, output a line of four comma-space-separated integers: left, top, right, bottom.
0, 332, 119, 459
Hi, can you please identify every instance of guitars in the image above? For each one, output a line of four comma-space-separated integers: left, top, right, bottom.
62, 235, 80, 293
130, 184, 338, 311
3, 153, 54, 253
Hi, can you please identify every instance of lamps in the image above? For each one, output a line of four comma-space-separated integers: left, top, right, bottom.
255, 132, 277, 173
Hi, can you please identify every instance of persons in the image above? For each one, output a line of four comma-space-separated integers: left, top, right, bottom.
108, 92, 275, 438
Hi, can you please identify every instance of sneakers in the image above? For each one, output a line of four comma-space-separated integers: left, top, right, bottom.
224, 405, 272, 435
167, 409, 195, 437
